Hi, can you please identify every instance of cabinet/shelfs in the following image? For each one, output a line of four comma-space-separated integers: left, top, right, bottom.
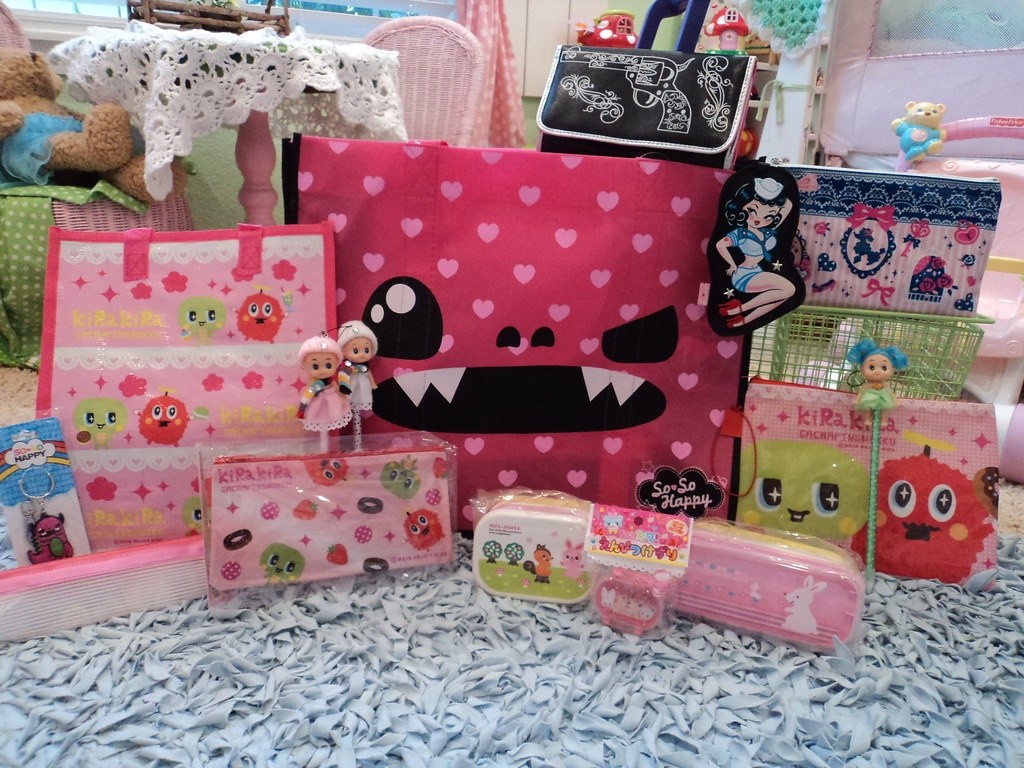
748, 39, 779, 109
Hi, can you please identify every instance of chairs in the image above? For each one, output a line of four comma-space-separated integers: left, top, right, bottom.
0, 2, 194, 231
364, 15, 486, 148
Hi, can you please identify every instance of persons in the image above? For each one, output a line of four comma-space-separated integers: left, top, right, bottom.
337, 320, 378, 412
847, 338, 907, 410
297, 336, 353, 433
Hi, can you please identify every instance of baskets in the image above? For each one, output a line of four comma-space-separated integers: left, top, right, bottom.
747, 307, 996, 403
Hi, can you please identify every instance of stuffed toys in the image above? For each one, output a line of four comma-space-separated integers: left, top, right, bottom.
0, 47, 187, 202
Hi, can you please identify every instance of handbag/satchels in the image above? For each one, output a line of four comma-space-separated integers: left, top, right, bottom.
536, 44, 759, 173
278, 130, 740, 526
33, 226, 342, 550
738, 379, 998, 596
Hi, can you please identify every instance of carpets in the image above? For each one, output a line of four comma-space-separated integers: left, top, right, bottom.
2, 479, 1024, 768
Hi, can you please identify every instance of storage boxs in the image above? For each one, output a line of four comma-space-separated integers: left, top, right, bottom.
768, 164, 1003, 318
473, 495, 863, 647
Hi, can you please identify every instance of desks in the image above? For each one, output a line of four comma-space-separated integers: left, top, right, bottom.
47, 19, 408, 225
